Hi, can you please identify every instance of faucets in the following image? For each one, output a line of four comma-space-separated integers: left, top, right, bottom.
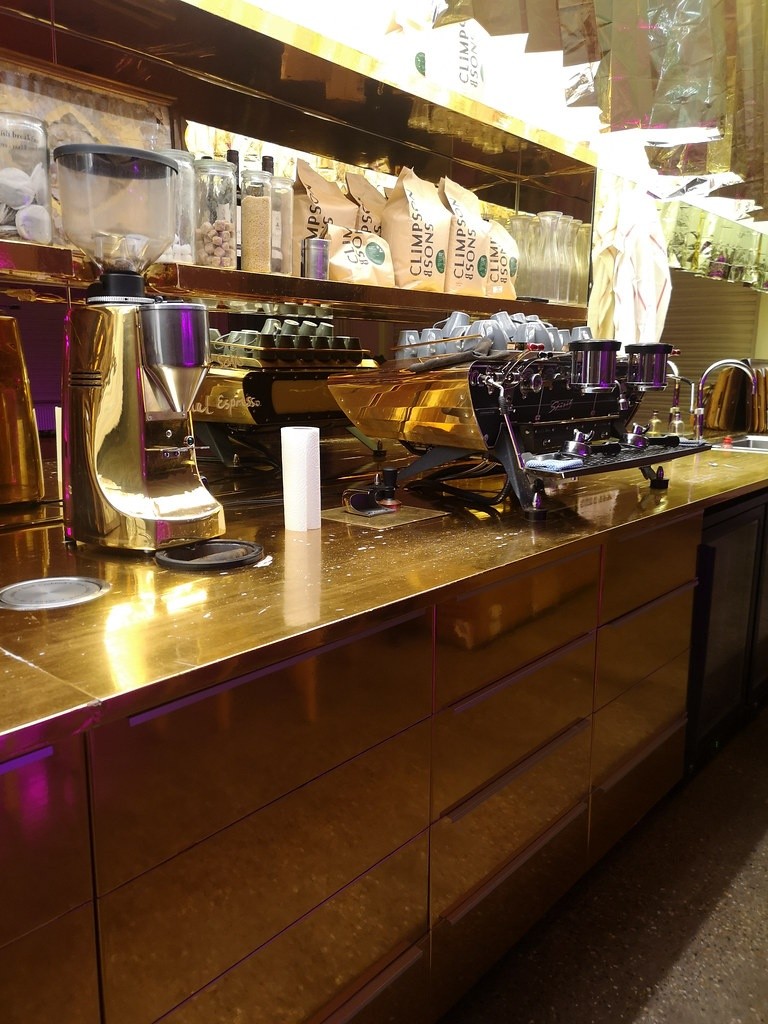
694, 359, 757, 440
665, 360, 681, 432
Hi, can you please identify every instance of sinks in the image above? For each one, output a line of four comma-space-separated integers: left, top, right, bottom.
711, 436, 768, 454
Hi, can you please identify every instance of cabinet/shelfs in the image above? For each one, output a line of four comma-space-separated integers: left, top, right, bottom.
685, 492, 768, 782
0, 0, 596, 320
0, 510, 703, 1024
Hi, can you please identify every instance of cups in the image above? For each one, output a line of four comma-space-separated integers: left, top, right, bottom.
395, 312, 594, 352
301, 238, 331, 281
209, 319, 363, 364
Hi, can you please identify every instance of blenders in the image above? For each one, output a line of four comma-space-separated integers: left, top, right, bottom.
55, 143, 225, 553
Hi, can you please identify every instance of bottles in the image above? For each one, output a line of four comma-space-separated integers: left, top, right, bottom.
478, 210, 591, 308
0, 112, 53, 247
153, 149, 196, 265
670, 411, 685, 438
271, 177, 294, 276
240, 170, 272, 275
648, 410, 662, 432
192, 160, 238, 270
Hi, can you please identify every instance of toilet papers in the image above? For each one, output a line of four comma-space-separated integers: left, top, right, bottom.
283, 532, 322, 627
280, 424, 322, 532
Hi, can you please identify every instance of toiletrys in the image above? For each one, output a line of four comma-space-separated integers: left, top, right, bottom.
648, 408, 685, 435
